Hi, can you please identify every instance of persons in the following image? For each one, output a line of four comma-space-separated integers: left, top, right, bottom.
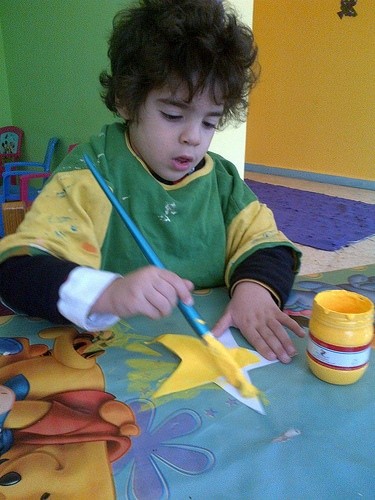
0, 0, 305, 364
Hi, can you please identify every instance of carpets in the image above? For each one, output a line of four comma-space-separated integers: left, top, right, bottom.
244, 178, 375, 251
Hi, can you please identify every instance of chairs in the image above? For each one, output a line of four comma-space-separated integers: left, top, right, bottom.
0, 126, 79, 238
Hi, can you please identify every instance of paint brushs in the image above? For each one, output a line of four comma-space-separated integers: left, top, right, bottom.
83, 152, 246, 387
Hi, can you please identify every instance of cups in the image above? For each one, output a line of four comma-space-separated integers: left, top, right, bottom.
304, 290, 375, 386
